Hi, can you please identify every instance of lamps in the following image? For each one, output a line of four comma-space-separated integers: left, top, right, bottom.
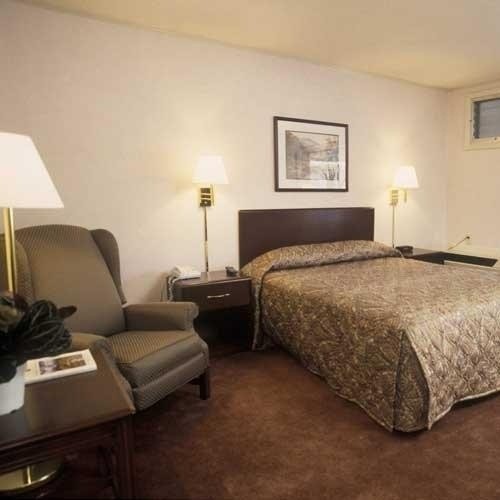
195, 151, 230, 278
0, 132, 67, 297
390, 162, 418, 246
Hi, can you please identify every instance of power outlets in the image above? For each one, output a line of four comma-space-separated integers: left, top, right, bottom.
464, 231, 473, 245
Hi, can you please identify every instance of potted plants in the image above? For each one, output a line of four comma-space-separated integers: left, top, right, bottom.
0, 293, 85, 414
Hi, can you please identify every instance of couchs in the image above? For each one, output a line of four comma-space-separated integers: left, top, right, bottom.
0, 222, 213, 430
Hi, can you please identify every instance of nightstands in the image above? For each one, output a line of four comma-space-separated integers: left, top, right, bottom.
168, 268, 253, 356
395, 245, 444, 265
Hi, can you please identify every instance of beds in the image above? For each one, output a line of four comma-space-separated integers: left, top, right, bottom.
235, 206, 500, 434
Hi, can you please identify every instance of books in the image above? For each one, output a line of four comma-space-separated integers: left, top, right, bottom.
24, 348, 98, 386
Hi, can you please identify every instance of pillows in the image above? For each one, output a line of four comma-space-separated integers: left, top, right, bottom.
263, 239, 400, 271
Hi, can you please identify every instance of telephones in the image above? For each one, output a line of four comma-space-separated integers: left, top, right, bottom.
172, 266, 201, 279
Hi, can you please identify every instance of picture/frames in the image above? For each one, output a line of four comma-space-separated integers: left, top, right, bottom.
271, 114, 350, 195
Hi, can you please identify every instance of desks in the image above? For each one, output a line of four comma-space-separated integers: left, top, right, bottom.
0, 343, 137, 500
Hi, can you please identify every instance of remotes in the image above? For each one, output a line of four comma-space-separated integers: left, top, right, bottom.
225, 266, 239, 274
